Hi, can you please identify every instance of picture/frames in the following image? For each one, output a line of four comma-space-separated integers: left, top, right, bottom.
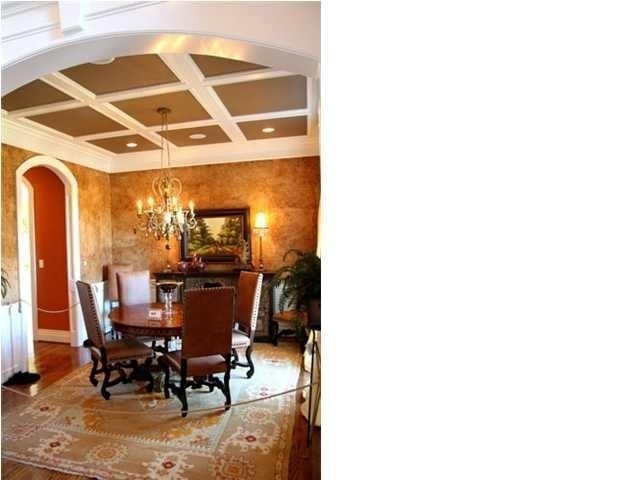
181, 209, 250, 263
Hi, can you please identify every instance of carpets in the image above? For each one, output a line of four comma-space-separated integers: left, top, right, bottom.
1, 340, 303, 480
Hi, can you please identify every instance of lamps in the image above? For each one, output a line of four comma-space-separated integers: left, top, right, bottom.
254, 215, 267, 268
162, 220, 174, 270
135, 108, 197, 250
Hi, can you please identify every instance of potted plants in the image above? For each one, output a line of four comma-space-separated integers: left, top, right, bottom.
267, 250, 321, 329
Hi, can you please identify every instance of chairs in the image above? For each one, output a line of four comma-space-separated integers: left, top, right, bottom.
208, 271, 263, 380
164, 287, 233, 418
117, 271, 168, 358
77, 281, 154, 400
